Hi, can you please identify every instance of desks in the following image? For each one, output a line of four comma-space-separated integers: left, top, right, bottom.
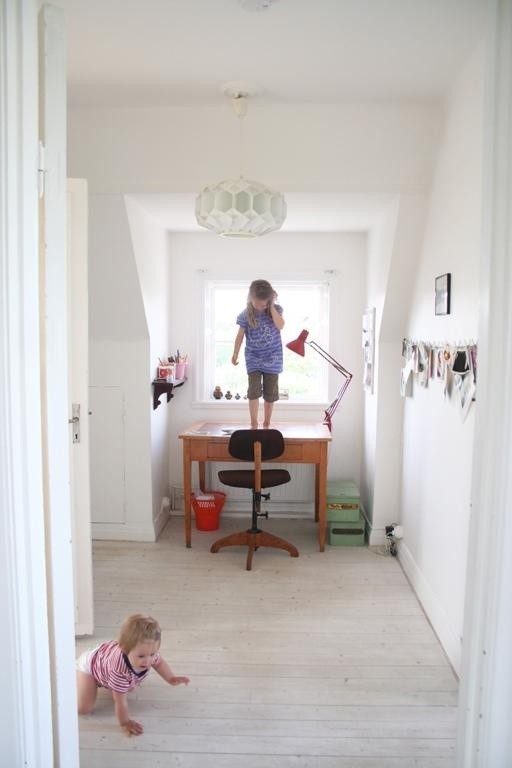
179, 421, 332, 553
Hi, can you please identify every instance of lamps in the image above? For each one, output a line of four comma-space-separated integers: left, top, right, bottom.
286, 330, 352, 432
195, 85, 288, 239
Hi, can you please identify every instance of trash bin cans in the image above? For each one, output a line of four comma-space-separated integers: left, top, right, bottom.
191, 491, 226, 530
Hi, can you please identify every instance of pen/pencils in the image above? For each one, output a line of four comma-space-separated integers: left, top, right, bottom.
158, 349, 189, 367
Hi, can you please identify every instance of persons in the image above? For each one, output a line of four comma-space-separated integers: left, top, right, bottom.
77, 613, 190, 736
231, 279, 285, 429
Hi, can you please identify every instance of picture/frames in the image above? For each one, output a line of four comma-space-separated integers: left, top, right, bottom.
434, 273, 451, 315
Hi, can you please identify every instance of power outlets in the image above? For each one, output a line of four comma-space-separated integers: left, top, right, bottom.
395, 526, 405, 539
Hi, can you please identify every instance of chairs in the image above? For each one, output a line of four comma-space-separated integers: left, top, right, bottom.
210, 428, 299, 571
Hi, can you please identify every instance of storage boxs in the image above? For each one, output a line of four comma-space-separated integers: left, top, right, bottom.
326, 479, 364, 547
